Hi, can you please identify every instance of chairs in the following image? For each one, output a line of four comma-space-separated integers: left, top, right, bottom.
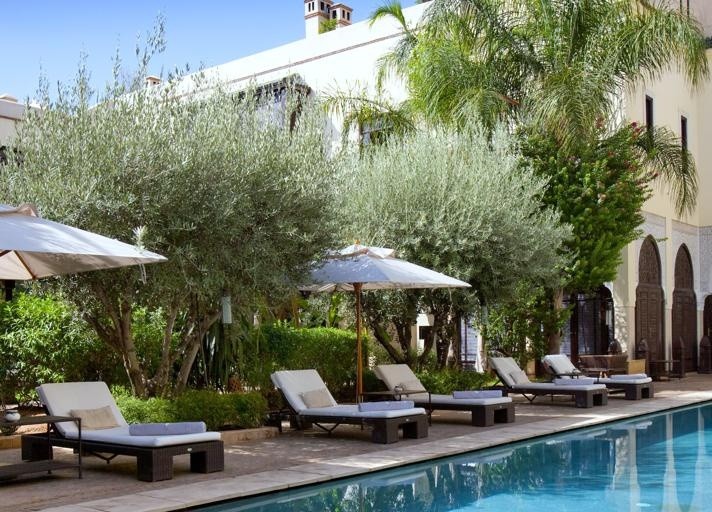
374, 364, 516, 426
20, 381, 224, 483
270, 369, 429, 446
542, 354, 652, 400
488, 358, 608, 409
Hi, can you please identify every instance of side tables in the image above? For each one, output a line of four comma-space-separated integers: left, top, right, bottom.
360, 390, 428, 401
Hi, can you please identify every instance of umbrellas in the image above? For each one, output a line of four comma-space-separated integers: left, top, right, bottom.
0, 196, 164, 281
280, 241, 471, 402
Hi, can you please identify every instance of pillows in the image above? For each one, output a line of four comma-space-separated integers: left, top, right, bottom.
298, 389, 333, 409
509, 370, 531, 385
69, 405, 121, 431
398, 380, 428, 397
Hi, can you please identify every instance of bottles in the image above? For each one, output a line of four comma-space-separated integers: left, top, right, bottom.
4, 409, 21, 422
394, 386, 402, 393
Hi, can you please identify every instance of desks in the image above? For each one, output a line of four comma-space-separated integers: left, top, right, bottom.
557, 372, 602, 383
